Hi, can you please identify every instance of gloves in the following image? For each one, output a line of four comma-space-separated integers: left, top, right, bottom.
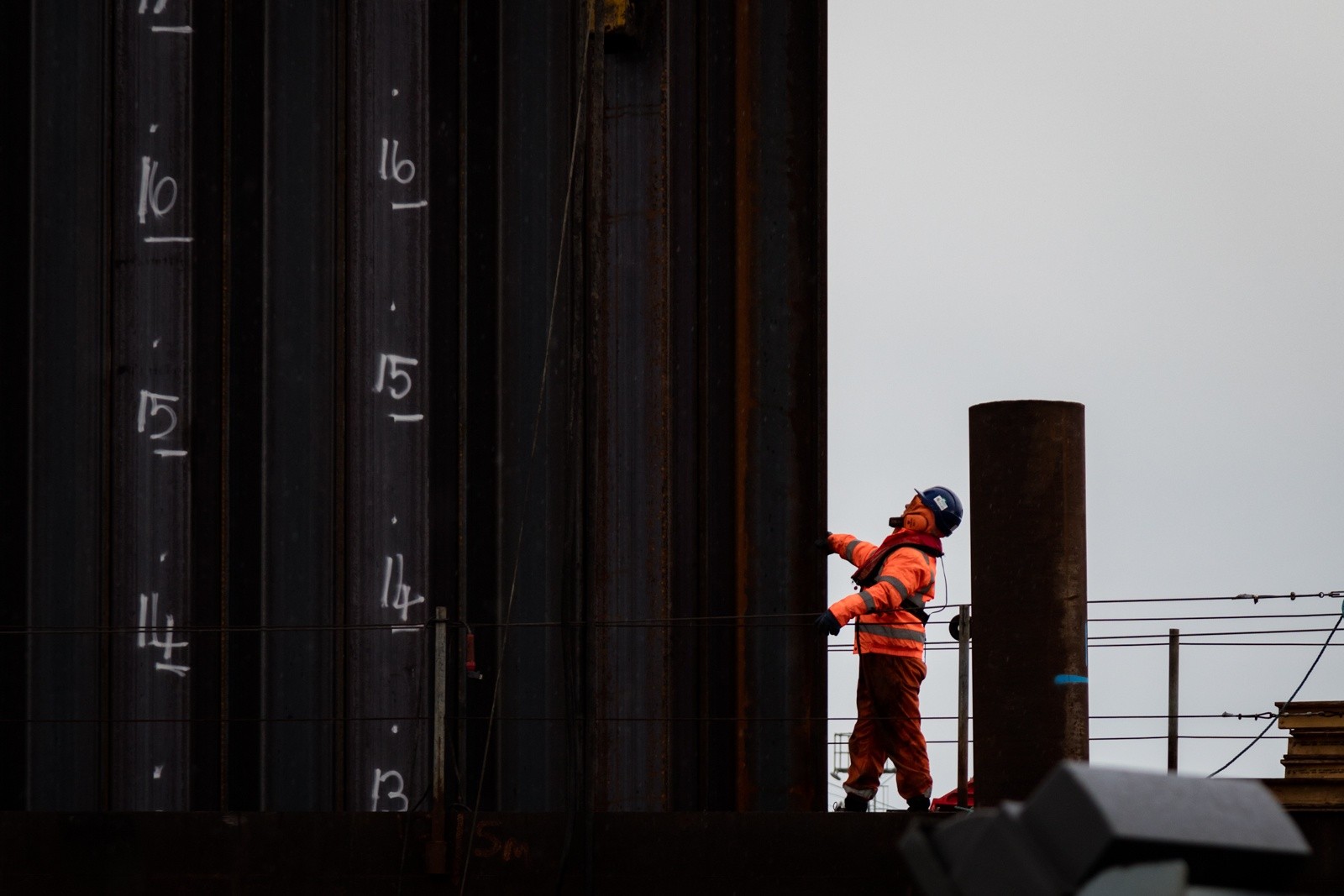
814, 611, 840, 636
827, 532, 833, 555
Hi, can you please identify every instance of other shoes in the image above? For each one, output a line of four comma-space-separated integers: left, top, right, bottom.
906, 805, 928, 813
833, 806, 869, 813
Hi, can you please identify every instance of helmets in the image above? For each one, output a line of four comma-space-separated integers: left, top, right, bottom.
914, 486, 963, 536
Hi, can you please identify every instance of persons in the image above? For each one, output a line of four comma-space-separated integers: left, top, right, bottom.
828, 485, 963, 812
